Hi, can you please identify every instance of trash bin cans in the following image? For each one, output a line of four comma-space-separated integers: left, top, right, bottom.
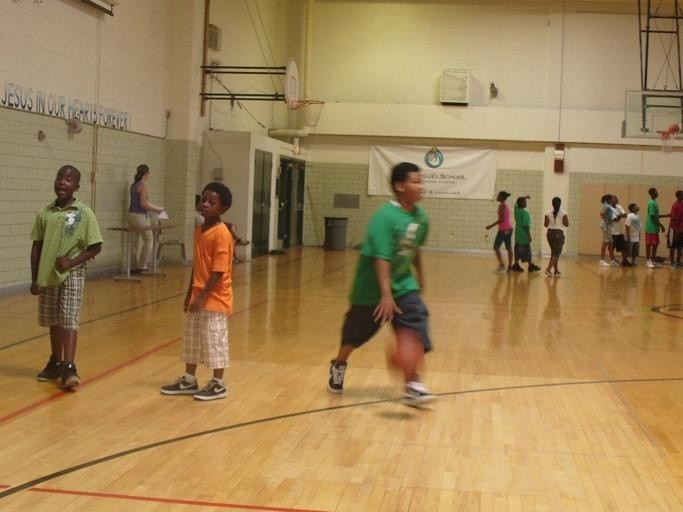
325, 216, 349, 250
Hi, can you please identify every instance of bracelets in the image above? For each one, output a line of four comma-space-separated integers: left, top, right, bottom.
66, 258, 75, 268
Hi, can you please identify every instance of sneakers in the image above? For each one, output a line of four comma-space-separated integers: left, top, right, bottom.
60, 362, 81, 389
402, 381, 438, 406
554, 271, 562, 277
512, 264, 524, 272
528, 264, 541, 272
494, 264, 506, 273
545, 269, 553, 277
328, 358, 348, 393
235, 238, 250, 246
598, 260, 611, 267
129, 267, 150, 274
192, 378, 228, 402
37, 354, 64, 382
610, 260, 620, 267
506, 265, 512, 273
620, 258, 683, 270
159, 376, 199, 395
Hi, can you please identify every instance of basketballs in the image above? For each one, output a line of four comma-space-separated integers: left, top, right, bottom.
669, 124, 678, 132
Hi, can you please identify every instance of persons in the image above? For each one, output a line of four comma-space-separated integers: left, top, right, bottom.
622, 203, 641, 266
193, 193, 250, 265
665, 190, 683, 268
609, 194, 631, 266
644, 187, 673, 269
124, 164, 164, 274
27, 162, 102, 390
326, 160, 436, 405
510, 194, 541, 272
159, 182, 234, 400
485, 190, 513, 273
543, 197, 569, 277
597, 194, 621, 267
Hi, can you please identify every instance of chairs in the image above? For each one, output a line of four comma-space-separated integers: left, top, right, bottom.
157, 229, 185, 266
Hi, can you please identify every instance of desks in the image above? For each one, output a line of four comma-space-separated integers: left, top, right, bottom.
107, 226, 173, 284
